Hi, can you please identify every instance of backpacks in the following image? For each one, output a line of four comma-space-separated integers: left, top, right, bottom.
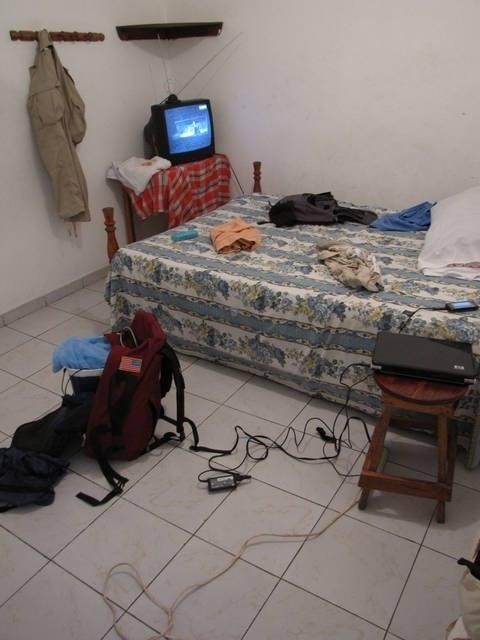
74, 309, 185, 508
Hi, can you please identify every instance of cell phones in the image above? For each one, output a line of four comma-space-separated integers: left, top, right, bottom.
446, 300, 478, 311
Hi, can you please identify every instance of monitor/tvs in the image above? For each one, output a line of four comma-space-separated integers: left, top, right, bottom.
144, 94, 215, 166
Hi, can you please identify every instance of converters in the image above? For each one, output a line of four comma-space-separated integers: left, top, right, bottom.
206, 472, 252, 492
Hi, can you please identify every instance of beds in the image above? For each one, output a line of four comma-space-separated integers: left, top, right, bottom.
103, 160, 480, 471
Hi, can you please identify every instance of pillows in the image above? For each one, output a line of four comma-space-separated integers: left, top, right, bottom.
415, 185, 480, 282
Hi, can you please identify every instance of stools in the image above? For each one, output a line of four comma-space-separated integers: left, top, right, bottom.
357, 372, 469, 525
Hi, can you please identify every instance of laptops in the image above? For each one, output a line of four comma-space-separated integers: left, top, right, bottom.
371, 332, 476, 386
